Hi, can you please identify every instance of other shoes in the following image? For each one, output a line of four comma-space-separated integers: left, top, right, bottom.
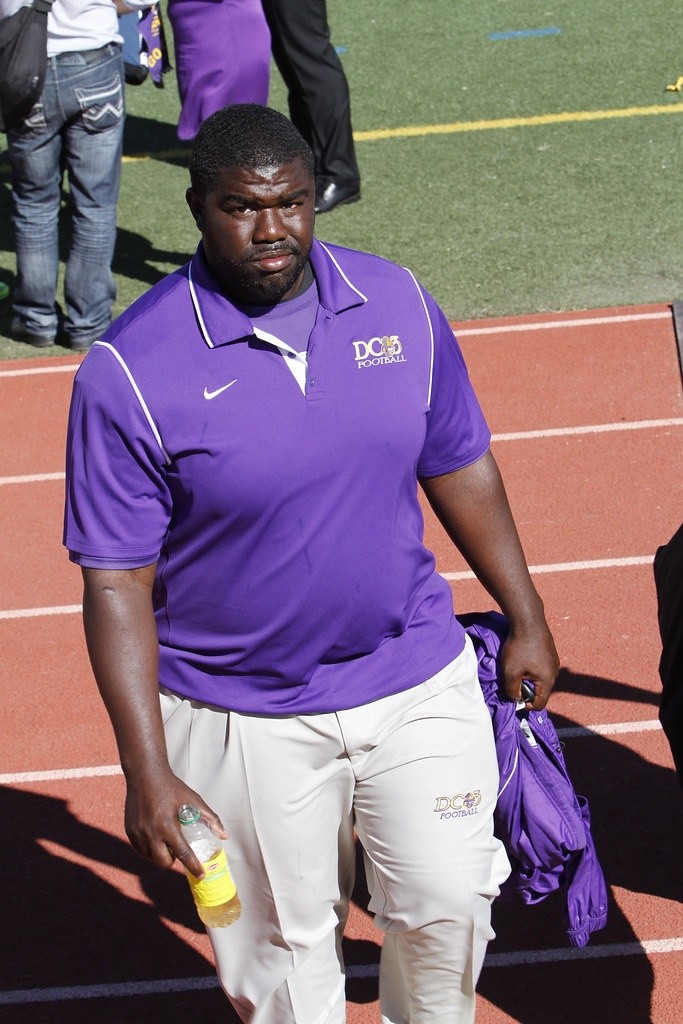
12, 333, 56, 347
72, 340, 94, 350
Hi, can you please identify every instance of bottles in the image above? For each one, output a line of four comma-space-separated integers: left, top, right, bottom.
179, 804, 241, 928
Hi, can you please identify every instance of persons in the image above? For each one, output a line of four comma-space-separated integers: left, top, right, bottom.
260, 1, 362, 214
63, 105, 560, 1024
0, 0, 159, 352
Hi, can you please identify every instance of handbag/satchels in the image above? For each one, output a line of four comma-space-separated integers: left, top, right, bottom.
0, 4, 48, 134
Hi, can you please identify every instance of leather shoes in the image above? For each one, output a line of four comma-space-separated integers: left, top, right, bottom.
314, 180, 360, 213
